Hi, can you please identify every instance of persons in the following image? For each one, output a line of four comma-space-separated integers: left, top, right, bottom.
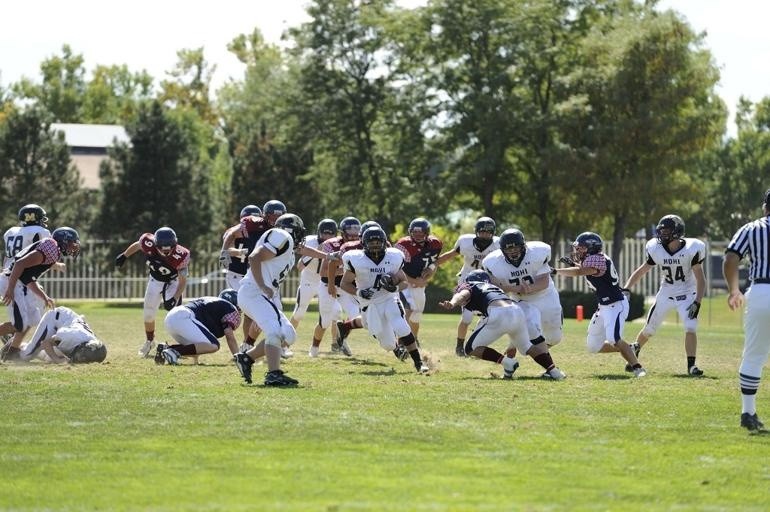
2, 204, 107, 365
721, 192, 769, 431
115, 200, 442, 388
620, 212, 710, 376
422, 217, 564, 379
546, 233, 648, 379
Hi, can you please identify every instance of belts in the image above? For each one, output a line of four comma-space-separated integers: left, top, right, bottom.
54, 313, 59, 330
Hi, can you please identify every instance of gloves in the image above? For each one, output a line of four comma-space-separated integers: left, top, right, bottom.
687, 301, 701, 320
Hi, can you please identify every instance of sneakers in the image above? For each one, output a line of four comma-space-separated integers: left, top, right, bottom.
0, 346, 26, 365
740, 410, 765, 436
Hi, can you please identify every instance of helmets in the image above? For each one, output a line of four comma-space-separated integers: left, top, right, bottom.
81, 340, 106, 363
18, 203, 686, 312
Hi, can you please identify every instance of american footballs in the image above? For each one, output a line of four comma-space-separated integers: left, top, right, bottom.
379, 274, 393, 286
